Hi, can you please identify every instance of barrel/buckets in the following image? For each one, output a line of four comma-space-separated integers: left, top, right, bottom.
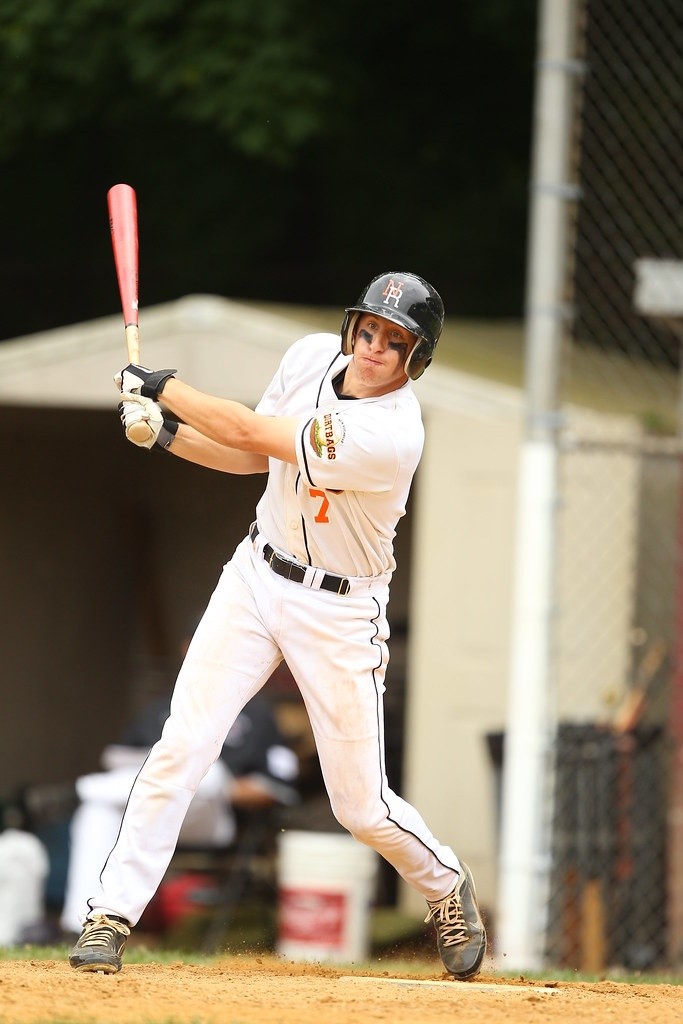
275, 831, 378, 965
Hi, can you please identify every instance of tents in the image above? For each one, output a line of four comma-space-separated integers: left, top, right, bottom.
0, 294, 680, 933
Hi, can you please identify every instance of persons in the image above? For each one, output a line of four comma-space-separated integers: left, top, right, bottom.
1, 728, 276, 950
66, 271, 488, 979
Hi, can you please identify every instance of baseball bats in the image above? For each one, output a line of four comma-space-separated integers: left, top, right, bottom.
104, 184, 151, 445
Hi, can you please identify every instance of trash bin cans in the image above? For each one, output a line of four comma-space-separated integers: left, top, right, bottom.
272, 829, 378, 969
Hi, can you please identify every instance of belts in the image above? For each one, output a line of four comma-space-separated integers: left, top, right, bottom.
250, 522, 350, 596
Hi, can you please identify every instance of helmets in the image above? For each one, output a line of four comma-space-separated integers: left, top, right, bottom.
341, 271, 445, 381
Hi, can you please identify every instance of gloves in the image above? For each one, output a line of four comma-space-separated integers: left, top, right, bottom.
118, 392, 179, 450
113, 363, 178, 403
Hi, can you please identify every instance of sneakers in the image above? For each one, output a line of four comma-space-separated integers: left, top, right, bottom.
69, 914, 131, 973
423, 859, 488, 981
